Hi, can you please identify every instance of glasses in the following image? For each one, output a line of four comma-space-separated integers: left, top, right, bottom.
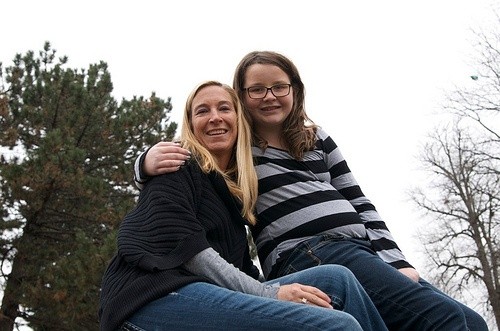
243, 83, 294, 99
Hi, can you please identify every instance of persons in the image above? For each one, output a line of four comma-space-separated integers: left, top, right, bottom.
98, 80, 389, 331
134, 51, 489, 331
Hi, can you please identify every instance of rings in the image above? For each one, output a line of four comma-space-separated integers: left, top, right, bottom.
301, 297, 307, 304
